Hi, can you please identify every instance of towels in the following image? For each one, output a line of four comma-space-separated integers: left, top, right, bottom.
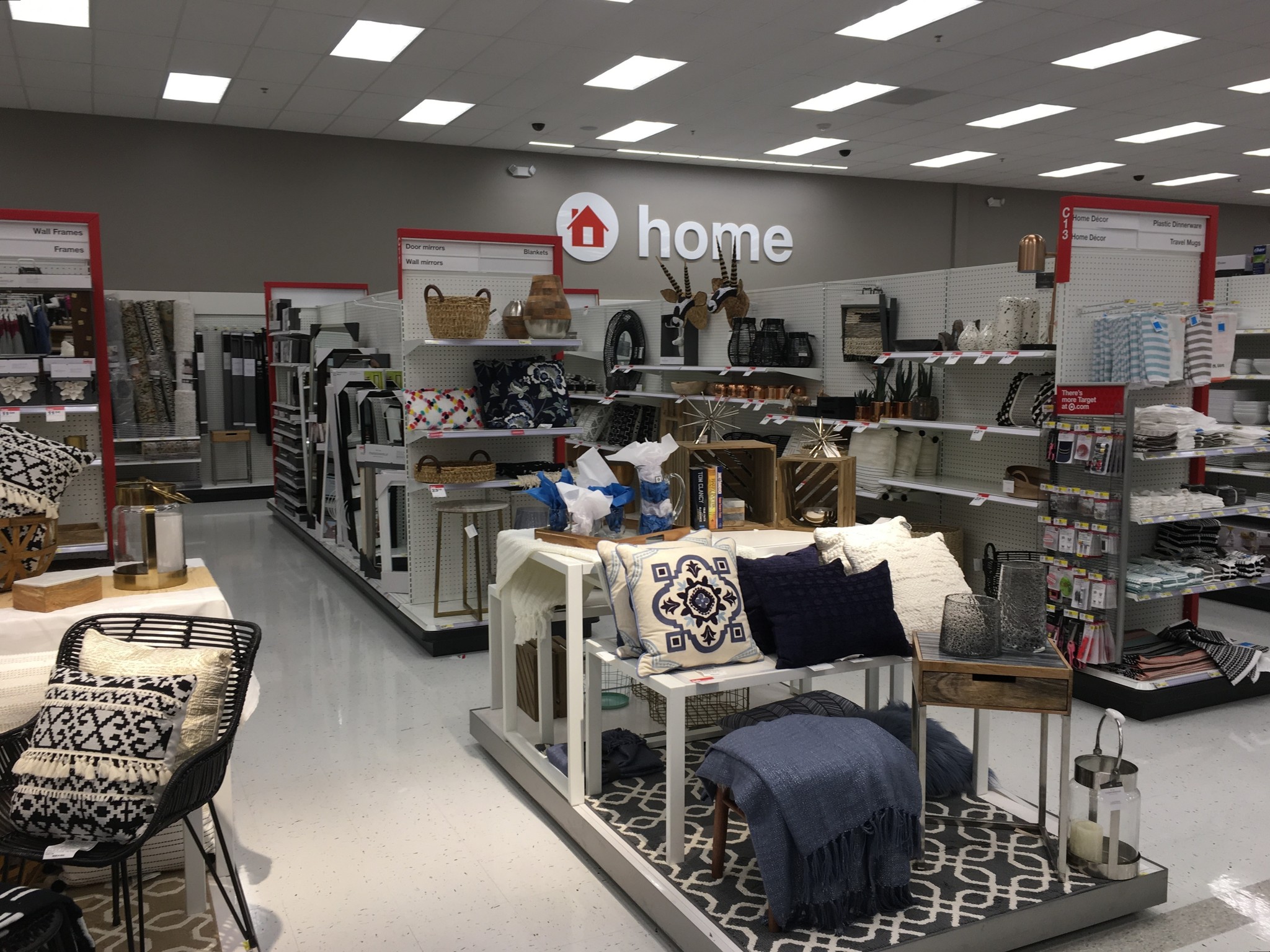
1100, 618, 1270, 686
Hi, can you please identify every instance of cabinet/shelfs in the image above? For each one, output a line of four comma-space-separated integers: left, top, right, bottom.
263, 229, 614, 657
0, 208, 205, 563
562, 197, 1270, 722
1199, 266, 1270, 616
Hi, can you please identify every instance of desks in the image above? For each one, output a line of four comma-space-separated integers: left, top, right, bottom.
586, 637, 915, 866
906, 627, 1075, 892
494, 521, 866, 809
487, 583, 615, 747
1, 556, 262, 917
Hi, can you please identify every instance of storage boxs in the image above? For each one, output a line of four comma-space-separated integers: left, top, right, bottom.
658, 313, 699, 367
776, 451, 857, 533
268, 298, 312, 522
667, 435, 778, 531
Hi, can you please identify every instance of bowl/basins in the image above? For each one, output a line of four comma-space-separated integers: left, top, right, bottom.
1230, 358, 1270, 375
801, 507, 836, 527
1209, 387, 1270, 425
670, 380, 707, 395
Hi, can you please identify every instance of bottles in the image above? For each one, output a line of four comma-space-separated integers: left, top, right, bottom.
502, 300, 529, 340
956, 296, 1041, 350
705, 382, 807, 400
522, 274, 572, 339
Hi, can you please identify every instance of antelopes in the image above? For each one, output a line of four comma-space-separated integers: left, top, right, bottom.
706, 234, 751, 332
655, 254, 708, 331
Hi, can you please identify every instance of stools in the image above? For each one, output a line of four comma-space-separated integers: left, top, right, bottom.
702, 737, 778, 932
430, 498, 510, 622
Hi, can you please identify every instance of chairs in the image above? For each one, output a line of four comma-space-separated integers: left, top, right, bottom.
1, 614, 263, 952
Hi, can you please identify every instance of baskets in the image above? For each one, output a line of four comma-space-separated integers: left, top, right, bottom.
908, 522, 963, 571
424, 283, 493, 339
415, 449, 497, 484
0, 513, 58, 594
981, 543, 1047, 599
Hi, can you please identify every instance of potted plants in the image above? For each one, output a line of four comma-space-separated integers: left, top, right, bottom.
853, 358, 940, 423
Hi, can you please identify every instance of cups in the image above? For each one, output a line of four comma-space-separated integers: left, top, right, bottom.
997, 559, 1048, 654
547, 506, 627, 538
938, 593, 1002, 657
635, 463, 685, 535
855, 396, 939, 422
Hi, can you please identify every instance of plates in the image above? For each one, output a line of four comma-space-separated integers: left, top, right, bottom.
1205, 455, 1270, 503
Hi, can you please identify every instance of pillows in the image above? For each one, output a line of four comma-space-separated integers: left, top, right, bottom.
401, 355, 662, 447
597, 513, 986, 682
782, 420, 944, 501
0, 423, 97, 574
9, 626, 216, 887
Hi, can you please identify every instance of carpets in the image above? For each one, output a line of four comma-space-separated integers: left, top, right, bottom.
1, 855, 223, 951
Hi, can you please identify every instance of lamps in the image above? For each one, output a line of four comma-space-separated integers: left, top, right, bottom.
1018, 232, 1059, 351
